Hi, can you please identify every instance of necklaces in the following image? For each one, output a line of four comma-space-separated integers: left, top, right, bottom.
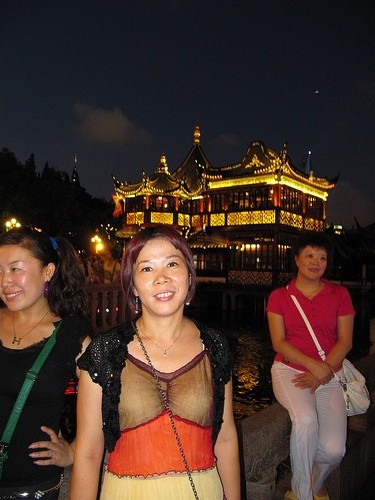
139, 316, 185, 358
300, 283, 321, 298
12, 309, 50, 345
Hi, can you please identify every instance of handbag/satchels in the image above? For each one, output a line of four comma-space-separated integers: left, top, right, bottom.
340, 358, 370, 416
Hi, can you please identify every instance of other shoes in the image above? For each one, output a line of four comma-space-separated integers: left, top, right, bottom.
314, 484, 330, 500
283, 490, 299, 500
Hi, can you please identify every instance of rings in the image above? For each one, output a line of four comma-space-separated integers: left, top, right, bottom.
51, 450, 54, 458
307, 384, 309, 388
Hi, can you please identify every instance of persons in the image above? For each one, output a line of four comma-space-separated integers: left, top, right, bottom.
0, 227, 91, 500
266, 234, 355, 500
68, 223, 241, 500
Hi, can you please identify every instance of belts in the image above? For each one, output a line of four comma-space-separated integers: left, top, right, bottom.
0, 472, 64, 500
